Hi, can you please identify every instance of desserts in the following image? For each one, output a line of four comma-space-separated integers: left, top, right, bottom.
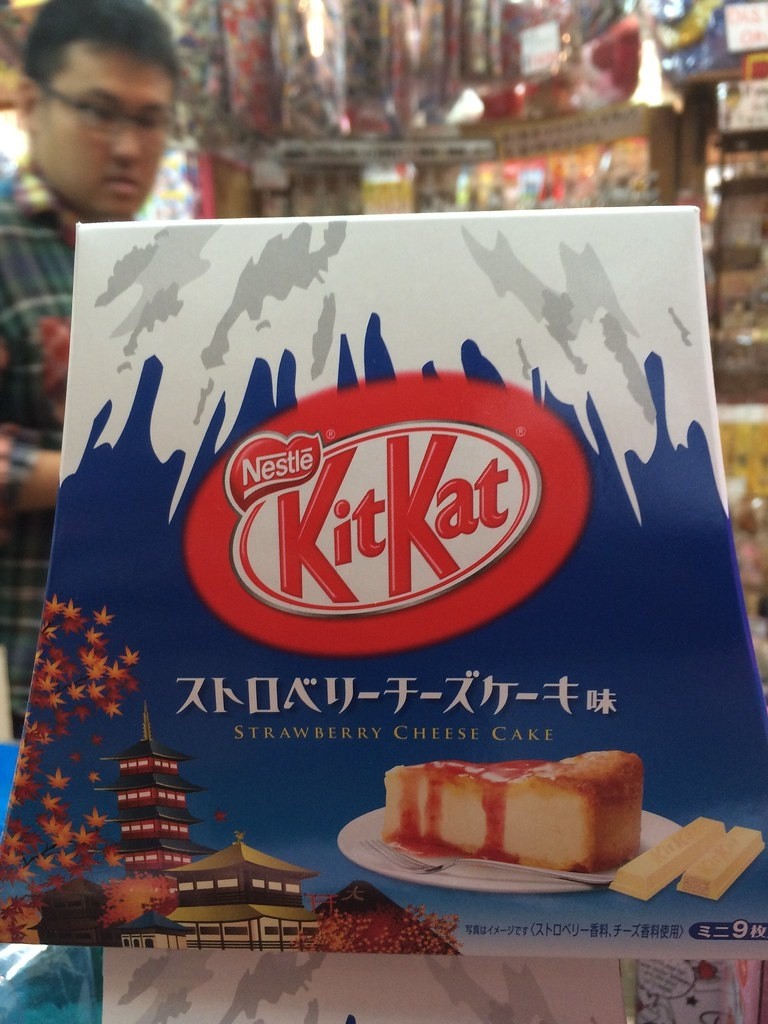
382, 750, 643, 873
607, 816, 765, 900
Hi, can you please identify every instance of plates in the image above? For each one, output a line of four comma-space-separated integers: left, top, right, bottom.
337, 807, 683, 894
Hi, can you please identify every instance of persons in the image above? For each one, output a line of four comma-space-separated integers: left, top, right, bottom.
1, 0, 179, 742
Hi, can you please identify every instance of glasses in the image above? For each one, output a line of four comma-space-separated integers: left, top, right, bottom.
37, 75, 180, 138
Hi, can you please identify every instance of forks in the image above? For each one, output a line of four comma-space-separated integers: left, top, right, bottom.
358, 839, 615, 884
